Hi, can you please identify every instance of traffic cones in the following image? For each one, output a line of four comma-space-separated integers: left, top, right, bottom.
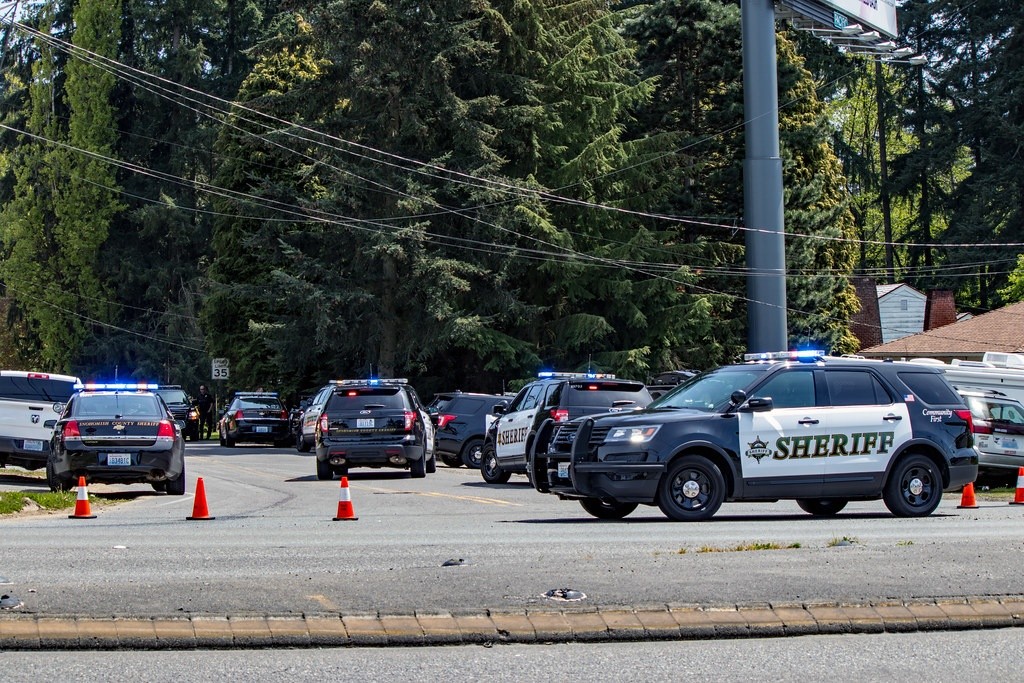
956, 481, 981, 509
331, 477, 360, 521
1009, 467, 1024, 504
68, 476, 99, 520
185, 476, 216, 520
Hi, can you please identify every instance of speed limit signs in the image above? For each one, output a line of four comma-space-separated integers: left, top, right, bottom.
211, 367, 232, 380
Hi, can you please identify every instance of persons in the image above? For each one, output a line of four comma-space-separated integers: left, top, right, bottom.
196, 385, 213, 440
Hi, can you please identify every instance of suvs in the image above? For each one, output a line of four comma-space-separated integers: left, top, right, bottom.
148, 383, 202, 442
218, 391, 293, 447
545, 351, 979, 522
314, 377, 437, 481
45, 382, 187, 497
426, 370, 701, 489
291, 387, 329, 453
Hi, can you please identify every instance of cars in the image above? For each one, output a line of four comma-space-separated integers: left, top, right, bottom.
951, 385, 1024, 489
1, 369, 83, 471
